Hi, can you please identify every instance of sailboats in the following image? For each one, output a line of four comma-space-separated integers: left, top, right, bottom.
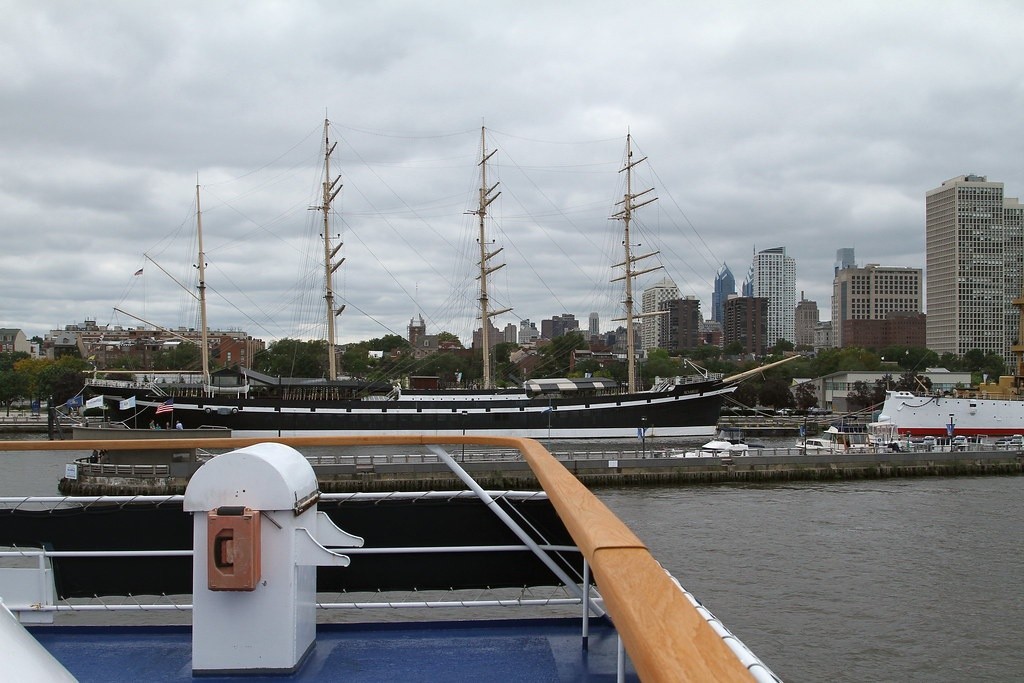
46, 108, 804, 444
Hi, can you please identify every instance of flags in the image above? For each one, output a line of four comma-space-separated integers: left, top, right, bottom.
155, 398, 173, 414
86, 394, 103, 409
67, 396, 82, 408
120, 396, 135, 410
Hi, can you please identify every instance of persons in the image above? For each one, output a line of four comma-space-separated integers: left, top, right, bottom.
176, 420, 184, 430
165, 422, 171, 429
156, 423, 161, 429
149, 420, 155, 429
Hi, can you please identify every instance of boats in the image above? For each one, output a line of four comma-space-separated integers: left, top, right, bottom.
658, 422, 906, 456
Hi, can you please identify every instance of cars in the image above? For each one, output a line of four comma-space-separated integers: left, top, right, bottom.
913, 433, 1024, 450
